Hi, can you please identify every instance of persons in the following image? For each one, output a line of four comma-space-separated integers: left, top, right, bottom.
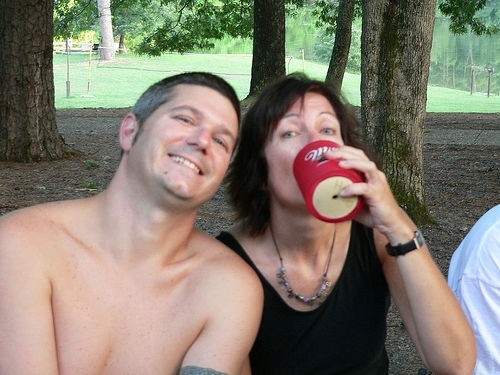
0, 71, 264, 375
447, 203, 500, 375
214, 74, 477, 375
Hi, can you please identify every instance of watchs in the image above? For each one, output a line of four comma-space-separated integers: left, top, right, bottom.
385, 229, 425, 257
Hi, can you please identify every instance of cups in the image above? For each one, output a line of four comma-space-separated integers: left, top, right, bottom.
292, 141, 366, 223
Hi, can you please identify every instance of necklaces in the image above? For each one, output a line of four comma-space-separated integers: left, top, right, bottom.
269, 217, 337, 303
